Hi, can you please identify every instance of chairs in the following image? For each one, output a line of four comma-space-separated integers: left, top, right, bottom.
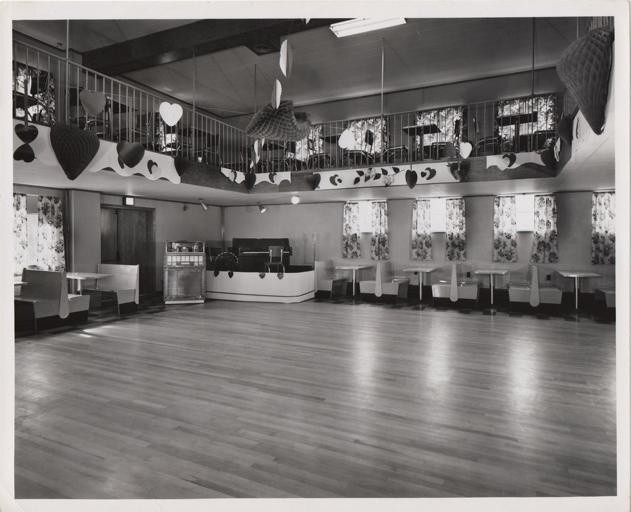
303, 111, 560, 154
14, 68, 223, 165
266, 246, 286, 272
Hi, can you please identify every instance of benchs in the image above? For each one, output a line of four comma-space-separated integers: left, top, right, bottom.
17, 264, 140, 330
316, 255, 616, 316
